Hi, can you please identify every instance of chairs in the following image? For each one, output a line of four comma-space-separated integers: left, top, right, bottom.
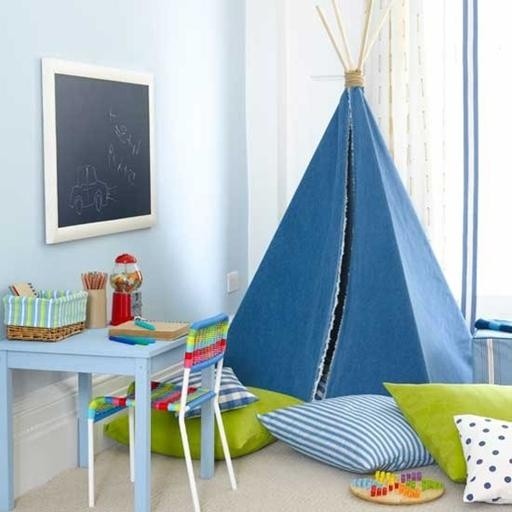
86, 311, 237, 512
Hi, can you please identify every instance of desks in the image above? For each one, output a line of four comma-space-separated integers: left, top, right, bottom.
0, 323, 216, 512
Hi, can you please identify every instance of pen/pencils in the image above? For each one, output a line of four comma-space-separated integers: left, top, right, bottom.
81, 271, 108, 290
135, 318, 156, 331
110, 334, 157, 345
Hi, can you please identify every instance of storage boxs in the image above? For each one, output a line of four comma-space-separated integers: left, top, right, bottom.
2, 287, 89, 343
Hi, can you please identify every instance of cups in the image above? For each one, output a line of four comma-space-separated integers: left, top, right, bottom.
84, 289, 107, 329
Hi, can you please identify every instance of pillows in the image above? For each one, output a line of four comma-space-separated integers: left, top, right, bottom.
163, 366, 260, 420
453, 414, 512, 505
382, 381, 512, 484
103, 385, 307, 461
256, 393, 438, 475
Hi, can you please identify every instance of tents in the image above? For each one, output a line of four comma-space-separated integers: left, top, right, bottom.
212, 0, 476, 402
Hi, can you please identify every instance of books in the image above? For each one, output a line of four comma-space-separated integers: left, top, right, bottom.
107, 318, 191, 341
5, 281, 38, 297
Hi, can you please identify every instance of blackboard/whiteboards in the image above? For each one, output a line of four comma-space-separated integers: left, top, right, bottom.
42, 56, 156, 245
